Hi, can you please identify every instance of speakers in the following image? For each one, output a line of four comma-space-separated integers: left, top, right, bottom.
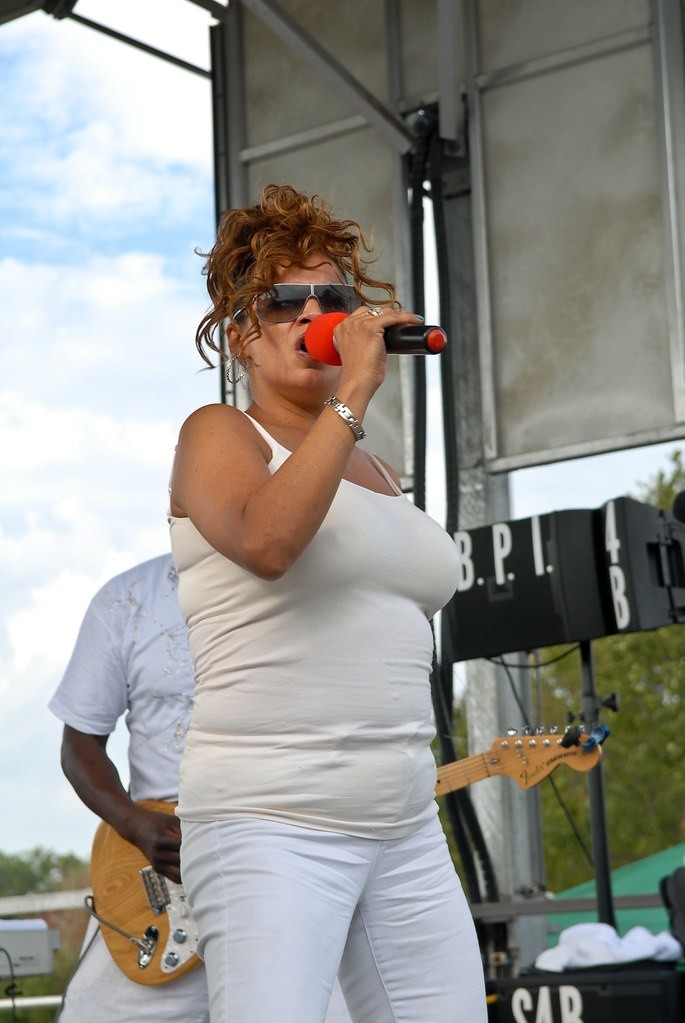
439, 495, 685, 663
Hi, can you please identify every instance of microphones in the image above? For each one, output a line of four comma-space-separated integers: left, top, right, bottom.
303, 313, 449, 366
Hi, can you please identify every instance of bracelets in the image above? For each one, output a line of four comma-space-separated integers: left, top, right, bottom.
323, 395, 366, 445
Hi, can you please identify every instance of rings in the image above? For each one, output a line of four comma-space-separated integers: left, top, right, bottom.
366, 307, 383, 315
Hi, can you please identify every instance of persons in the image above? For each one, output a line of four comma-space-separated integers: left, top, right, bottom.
167, 181, 490, 1023
47, 551, 352, 1023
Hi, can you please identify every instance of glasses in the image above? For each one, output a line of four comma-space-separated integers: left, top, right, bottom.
233, 284, 363, 323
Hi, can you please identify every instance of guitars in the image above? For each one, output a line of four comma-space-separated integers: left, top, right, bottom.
89, 713, 612, 989
